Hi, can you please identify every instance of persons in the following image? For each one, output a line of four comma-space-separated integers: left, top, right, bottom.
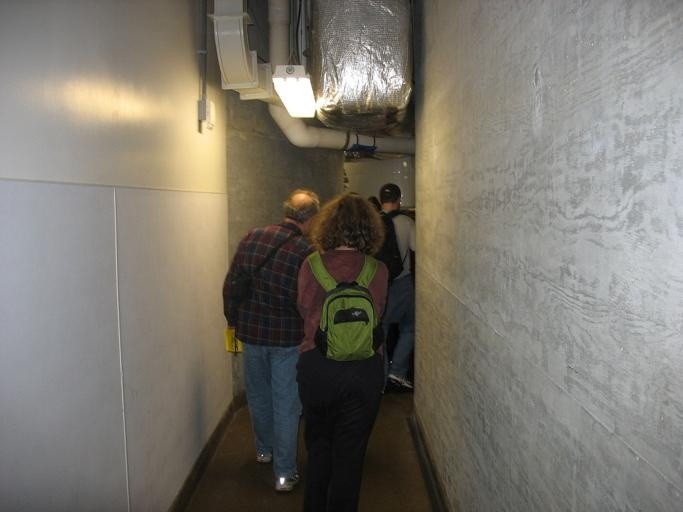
222, 188, 320, 492
295, 191, 389, 511
367, 196, 382, 213
378, 183, 416, 390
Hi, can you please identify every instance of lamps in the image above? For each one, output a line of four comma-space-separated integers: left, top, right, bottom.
272, 64, 316, 118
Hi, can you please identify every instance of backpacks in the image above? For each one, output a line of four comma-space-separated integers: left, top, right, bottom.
376, 208, 405, 280
223, 269, 251, 329
313, 283, 385, 365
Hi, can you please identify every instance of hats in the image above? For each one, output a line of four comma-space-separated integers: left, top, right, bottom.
380, 183, 400, 203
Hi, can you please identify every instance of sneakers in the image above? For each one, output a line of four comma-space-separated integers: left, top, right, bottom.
388, 372, 414, 391
274, 471, 302, 492
255, 452, 274, 464
